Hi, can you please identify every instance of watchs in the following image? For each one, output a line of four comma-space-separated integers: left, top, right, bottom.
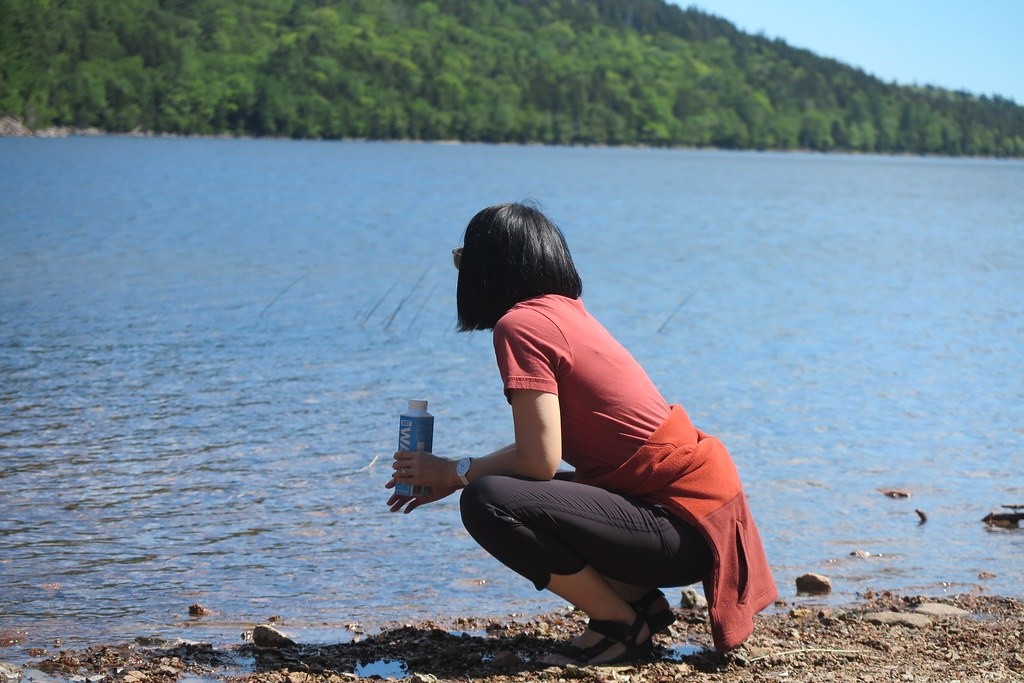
456, 457, 473, 486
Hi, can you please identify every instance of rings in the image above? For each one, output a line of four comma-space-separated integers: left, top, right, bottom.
404, 468, 408, 478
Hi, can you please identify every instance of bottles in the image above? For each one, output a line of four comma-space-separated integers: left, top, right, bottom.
395, 399, 434, 497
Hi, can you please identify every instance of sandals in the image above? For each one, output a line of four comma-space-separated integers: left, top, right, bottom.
622, 590, 676, 631
535, 612, 653, 670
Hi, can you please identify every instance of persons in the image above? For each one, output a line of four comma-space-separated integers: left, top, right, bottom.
385, 203, 777, 668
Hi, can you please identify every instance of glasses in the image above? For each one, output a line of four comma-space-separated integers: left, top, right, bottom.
452, 247, 463, 269
